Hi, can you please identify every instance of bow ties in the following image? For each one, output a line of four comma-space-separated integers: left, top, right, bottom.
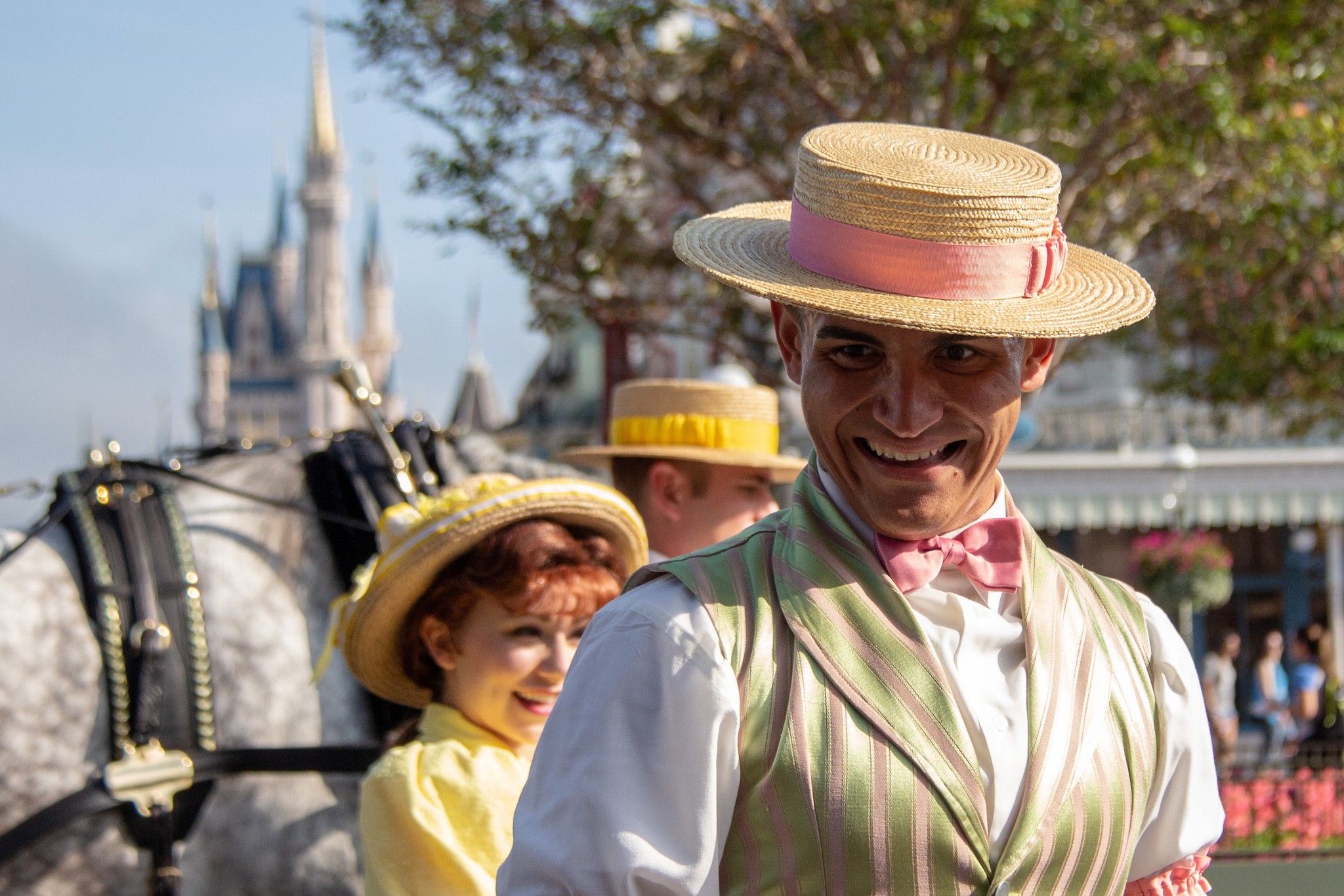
872, 517, 1026, 598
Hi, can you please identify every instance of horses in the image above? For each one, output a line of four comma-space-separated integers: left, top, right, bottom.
2, 422, 609, 896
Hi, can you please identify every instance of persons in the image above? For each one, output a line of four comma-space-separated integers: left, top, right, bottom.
332, 474, 649, 896
1202, 621, 1334, 784
556, 378, 810, 567
495, 123, 1225, 896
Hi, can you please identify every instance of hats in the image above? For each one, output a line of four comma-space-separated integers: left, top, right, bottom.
558, 379, 808, 483
310, 471, 651, 713
673, 120, 1157, 346
